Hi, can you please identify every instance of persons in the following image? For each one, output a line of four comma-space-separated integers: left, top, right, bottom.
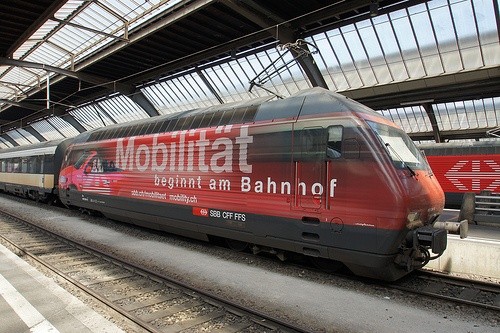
87, 159, 114, 173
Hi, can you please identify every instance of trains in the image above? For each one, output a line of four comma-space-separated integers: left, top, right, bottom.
417, 140, 499, 210
0, 90, 447, 284
